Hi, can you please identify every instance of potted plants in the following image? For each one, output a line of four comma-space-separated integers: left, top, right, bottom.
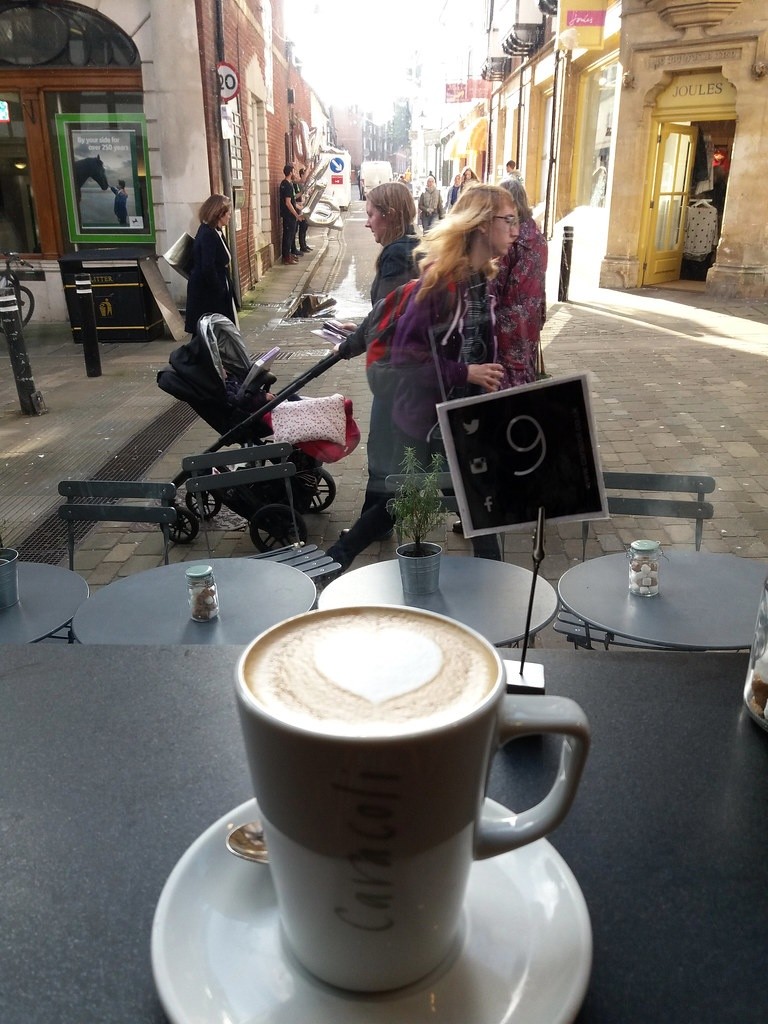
385, 446, 446, 595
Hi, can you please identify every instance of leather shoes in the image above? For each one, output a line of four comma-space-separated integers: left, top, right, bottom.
339, 527, 395, 541
453, 521, 462, 533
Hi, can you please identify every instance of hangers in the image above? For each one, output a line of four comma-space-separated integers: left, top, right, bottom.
690, 198, 712, 209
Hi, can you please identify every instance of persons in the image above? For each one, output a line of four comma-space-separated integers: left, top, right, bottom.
280, 166, 313, 264
320, 184, 518, 589
220, 357, 302, 411
444, 168, 479, 213
506, 160, 523, 184
109, 180, 128, 224
418, 172, 444, 228
185, 195, 240, 340
398, 176, 407, 184
453, 180, 548, 533
357, 171, 362, 200
334, 180, 423, 538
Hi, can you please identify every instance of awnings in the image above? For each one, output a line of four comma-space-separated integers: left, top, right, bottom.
444, 118, 487, 160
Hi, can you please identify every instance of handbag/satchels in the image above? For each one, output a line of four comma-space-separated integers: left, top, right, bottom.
163, 231, 195, 280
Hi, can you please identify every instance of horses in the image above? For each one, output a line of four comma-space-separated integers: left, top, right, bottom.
74, 154, 109, 202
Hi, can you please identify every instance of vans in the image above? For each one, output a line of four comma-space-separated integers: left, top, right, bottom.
358, 160, 393, 201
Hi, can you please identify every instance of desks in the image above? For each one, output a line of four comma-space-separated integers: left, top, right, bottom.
72, 558, 317, 644
0, 561, 90, 645
0, 642, 768, 1024
558, 550, 768, 653
317, 556, 559, 648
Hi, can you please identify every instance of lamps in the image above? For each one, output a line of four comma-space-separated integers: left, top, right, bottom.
621, 70, 634, 88
753, 62, 767, 81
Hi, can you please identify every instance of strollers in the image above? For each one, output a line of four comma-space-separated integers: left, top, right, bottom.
154, 309, 368, 552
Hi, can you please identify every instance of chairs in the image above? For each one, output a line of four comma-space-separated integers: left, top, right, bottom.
58, 480, 176, 645
552, 472, 715, 652
384, 471, 505, 564
182, 441, 342, 594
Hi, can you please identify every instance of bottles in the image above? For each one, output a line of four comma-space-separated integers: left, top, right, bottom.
186, 565, 219, 624
743, 579, 768, 731
628, 540, 658, 597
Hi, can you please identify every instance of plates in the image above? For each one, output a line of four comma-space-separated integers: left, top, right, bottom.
148, 792, 592, 1023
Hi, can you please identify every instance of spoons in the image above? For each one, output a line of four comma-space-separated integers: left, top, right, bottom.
225, 820, 268, 865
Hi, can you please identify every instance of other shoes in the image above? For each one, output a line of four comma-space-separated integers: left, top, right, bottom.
282, 246, 314, 265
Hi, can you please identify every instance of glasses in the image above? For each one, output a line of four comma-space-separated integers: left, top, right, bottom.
495, 215, 520, 224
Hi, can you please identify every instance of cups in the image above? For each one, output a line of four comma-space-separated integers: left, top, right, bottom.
0, 548, 20, 610
233, 603, 590, 997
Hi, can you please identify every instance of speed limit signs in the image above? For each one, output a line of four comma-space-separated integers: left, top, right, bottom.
217, 61, 241, 103
